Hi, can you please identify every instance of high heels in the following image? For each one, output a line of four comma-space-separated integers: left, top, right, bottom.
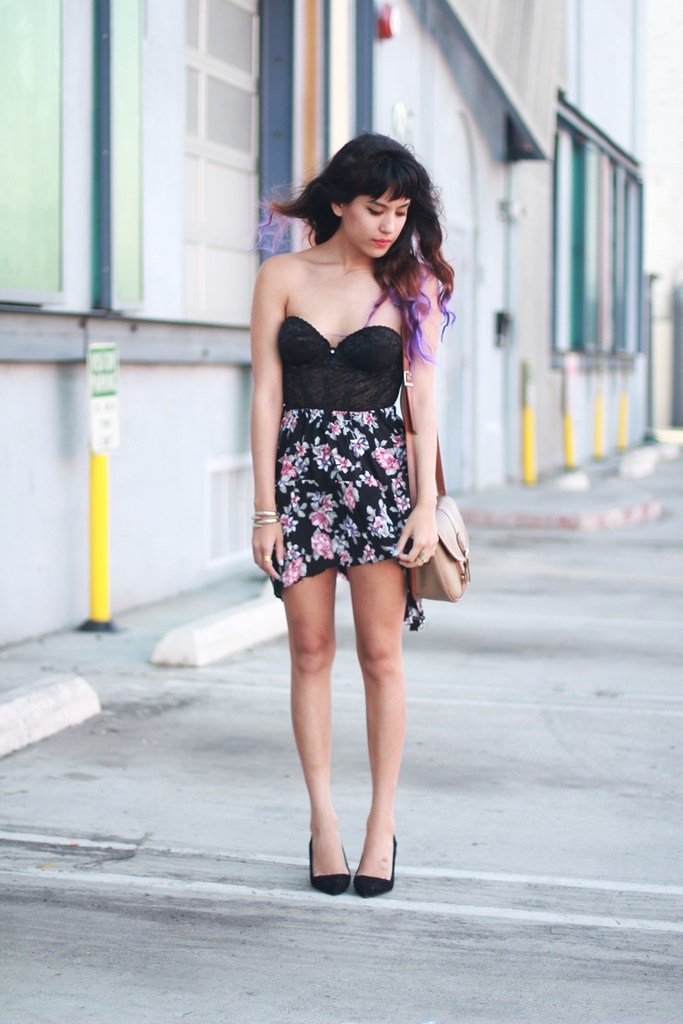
309, 837, 352, 897
353, 834, 397, 899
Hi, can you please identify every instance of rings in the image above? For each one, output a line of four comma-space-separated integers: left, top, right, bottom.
419, 555, 427, 564
262, 555, 270, 561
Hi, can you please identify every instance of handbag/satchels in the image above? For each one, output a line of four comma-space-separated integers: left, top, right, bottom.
409, 494, 471, 603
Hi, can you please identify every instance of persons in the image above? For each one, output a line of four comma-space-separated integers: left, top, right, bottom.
251, 134, 452, 899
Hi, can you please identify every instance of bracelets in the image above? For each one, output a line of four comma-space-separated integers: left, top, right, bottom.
251, 510, 280, 528
